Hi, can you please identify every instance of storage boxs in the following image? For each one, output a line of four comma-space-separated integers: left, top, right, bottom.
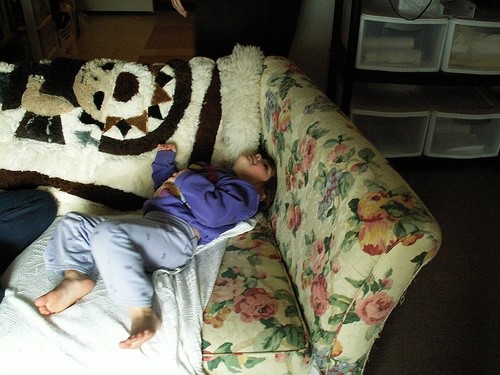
442, 17, 500, 76
350, 0, 449, 73
423, 85, 500, 159
349, 81, 431, 159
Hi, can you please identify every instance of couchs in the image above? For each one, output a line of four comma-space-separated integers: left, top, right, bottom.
0, 55, 441, 375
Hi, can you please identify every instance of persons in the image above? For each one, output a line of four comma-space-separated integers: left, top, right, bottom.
0, 190, 58, 303
32, 143, 278, 349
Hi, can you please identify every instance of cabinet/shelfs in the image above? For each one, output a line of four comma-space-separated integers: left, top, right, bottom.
7, 0, 78, 53
325, 0, 500, 163
